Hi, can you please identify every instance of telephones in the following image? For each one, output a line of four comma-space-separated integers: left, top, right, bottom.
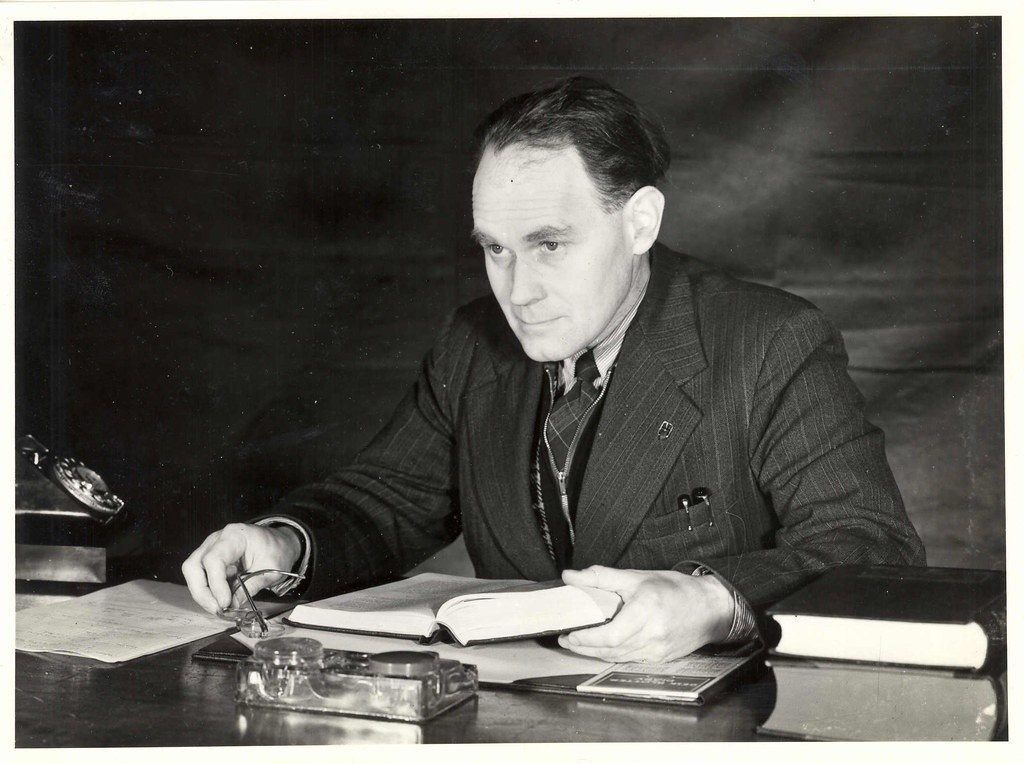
15, 432, 125, 596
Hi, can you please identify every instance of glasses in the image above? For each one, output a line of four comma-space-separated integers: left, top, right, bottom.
216, 569, 306, 639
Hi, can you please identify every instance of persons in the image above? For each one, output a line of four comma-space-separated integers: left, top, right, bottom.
180, 73, 930, 670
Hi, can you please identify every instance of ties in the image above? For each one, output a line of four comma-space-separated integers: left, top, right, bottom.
545, 349, 603, 472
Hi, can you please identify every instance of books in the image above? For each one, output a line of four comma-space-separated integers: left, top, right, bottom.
765, 561, 1008, 675
281, 569, 625, 647
751, 660, 1008, 741
